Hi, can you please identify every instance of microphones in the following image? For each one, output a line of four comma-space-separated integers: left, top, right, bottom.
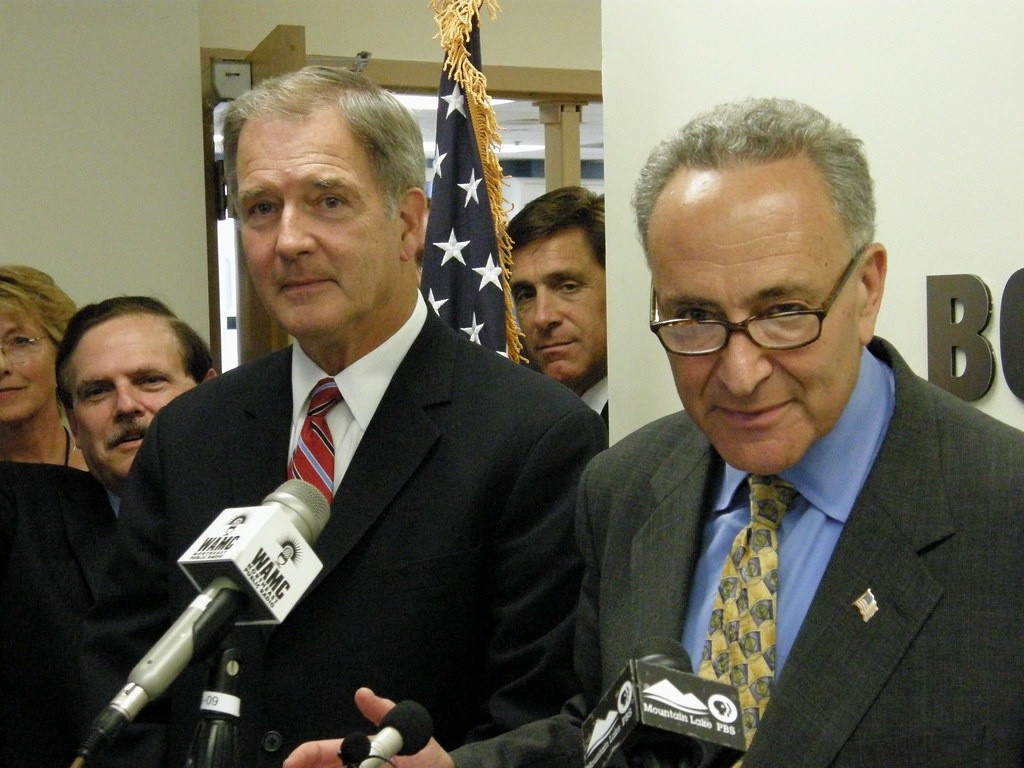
73, 479, 333, 762
582, 636, 747, 768
337, 699, 434, 768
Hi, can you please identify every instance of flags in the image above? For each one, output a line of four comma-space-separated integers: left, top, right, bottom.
416, 0, 543, 372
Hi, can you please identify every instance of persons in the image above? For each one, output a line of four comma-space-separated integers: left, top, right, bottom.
54, 298, 219, 518
282, 98, 1024, 768
505, 184, 608, 420
0, 460, 117, 768
60, 71, 609, 768
0, 265, 92, 476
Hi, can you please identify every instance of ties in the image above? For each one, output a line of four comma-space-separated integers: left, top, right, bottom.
288, 378, 344, 505
698, 474, 797, 768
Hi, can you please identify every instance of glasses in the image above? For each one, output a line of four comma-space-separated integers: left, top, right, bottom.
0, 334, 52, 347
649, 243, 866, 356
